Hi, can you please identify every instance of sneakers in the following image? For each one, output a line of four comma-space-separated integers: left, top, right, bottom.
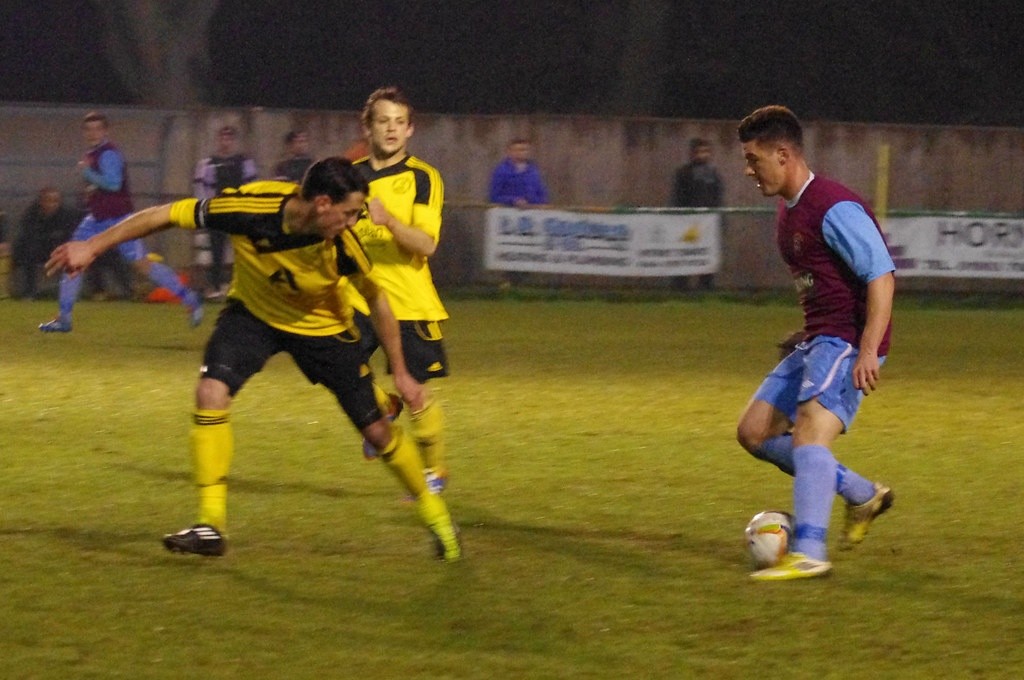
38, 316, 73, 332
163, 523, 226, 556
837, 482, 894, 552
190, 292, 205, 326
405, 465, 447, 501
749, 552, 833, 581
362, 393, 403, 459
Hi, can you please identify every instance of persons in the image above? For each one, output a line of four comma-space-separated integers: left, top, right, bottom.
351, 88, 448, 496
487, 137, 551, 291
672, 138, 724, 296
6, 125, 315, 299
45, 157, 464, 565
38, 112, 204, 332
736, 107, 896, 584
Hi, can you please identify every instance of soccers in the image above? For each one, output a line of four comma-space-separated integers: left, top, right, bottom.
745, 510, 795, 566
